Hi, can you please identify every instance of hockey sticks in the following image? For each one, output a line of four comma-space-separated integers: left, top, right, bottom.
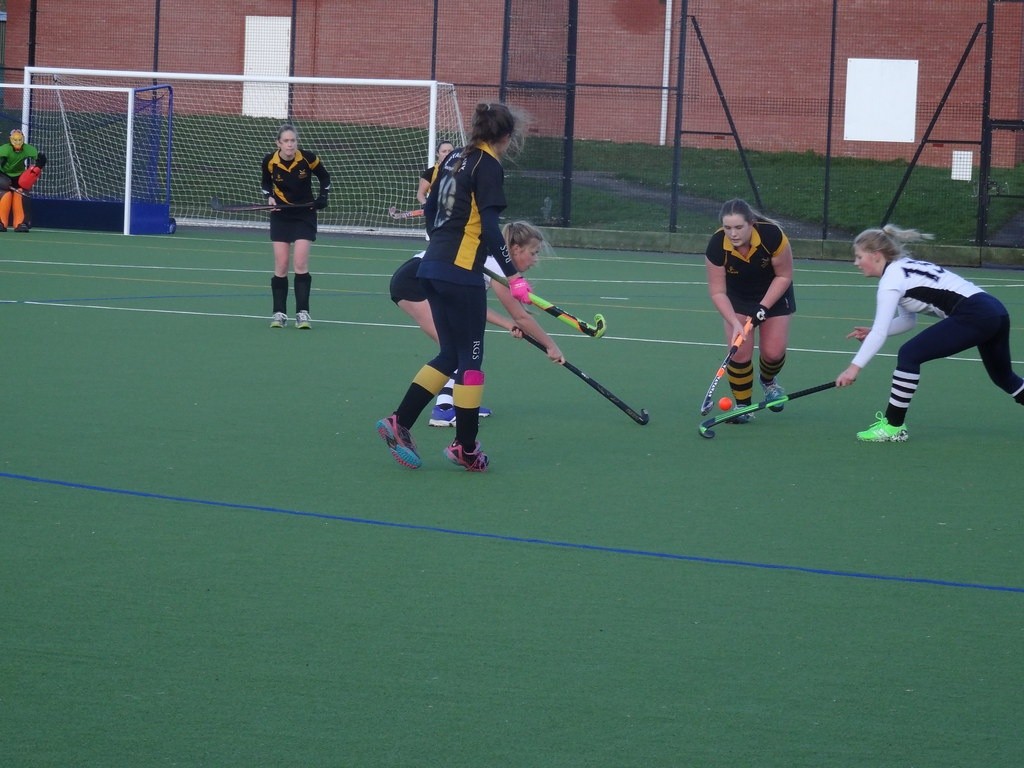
698, 377, 857, 439
511, 325, 650, 426
483, 266, 607, 339
210, 196, 316, 212
389, 206, 424, 220
700, 316, 755, 417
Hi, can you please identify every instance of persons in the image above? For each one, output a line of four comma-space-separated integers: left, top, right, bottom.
417, 141, 455, 210
0, 129, 47, 232
390, 221, 565, 427
836, 224, 1024, 443
705, 199, 796, 424
261, 125, 330, 329
377, 103, 532, 472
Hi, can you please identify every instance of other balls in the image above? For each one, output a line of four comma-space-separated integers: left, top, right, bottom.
718, 397, 733, 411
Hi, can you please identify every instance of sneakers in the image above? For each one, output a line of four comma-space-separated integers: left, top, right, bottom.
857, 411, 908, 443
726, 404, 754, 424
478, 407, 490, 416
271, 312, 288, 327
444, 437, 490, 472
295, 310, 312, 329
757, 373, 785, 412
429, 406, 479, 428
377, 412, 421, 469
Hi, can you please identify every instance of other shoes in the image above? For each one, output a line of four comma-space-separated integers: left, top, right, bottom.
0, 222, 7, 232
15, 224, 29, 232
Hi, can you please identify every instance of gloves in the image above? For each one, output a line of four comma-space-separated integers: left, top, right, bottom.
508, 276, 534, 305
309, 194, 329, 211
